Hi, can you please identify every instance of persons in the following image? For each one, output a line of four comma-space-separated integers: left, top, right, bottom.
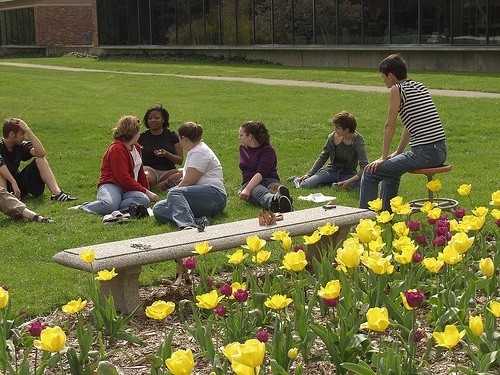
0, 187, 53, 224
288, 112, 370, 188
68, 115, 159, 221
0, 118, 78, 202
152, 123, 228, 230
137, 105, 184, 191
237, 120, 294, 212
358, 54, 447, 225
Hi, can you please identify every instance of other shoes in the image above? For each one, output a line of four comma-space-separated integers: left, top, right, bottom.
196, 216, 211, 226
286, 176, 296, 183
278, 185, 293, 212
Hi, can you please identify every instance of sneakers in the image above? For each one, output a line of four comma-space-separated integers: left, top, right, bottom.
51, 190, 76, 202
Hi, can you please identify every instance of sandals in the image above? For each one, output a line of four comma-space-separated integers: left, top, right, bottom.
27, 214, 55, 223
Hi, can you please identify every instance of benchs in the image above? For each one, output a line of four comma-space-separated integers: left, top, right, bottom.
52, 204, 379, 314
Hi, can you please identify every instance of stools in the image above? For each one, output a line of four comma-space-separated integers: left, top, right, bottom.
406, 163, 459, 223
